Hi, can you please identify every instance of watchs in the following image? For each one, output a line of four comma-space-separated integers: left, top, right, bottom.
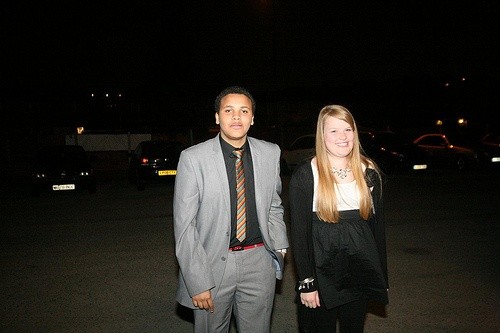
276, 249, 287, 253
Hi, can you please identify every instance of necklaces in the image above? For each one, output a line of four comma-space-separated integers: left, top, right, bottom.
330, 165, 351, 179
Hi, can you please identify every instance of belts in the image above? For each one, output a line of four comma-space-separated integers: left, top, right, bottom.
229, 243, 263, 251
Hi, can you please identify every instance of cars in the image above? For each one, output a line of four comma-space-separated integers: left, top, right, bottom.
129, 141, 171, 173
34, 143, 99, 195
408, 132, 473, 155
356, 130, 387, 163
281, 134, 318, 169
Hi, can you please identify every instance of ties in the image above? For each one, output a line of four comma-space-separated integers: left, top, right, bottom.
231, 149, 246, 243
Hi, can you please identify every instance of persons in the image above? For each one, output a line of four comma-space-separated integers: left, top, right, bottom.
289, 105, 389, 333
173, 85, 290, 333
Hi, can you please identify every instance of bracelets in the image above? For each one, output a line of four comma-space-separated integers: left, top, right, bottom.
298, 276, 314, 290
